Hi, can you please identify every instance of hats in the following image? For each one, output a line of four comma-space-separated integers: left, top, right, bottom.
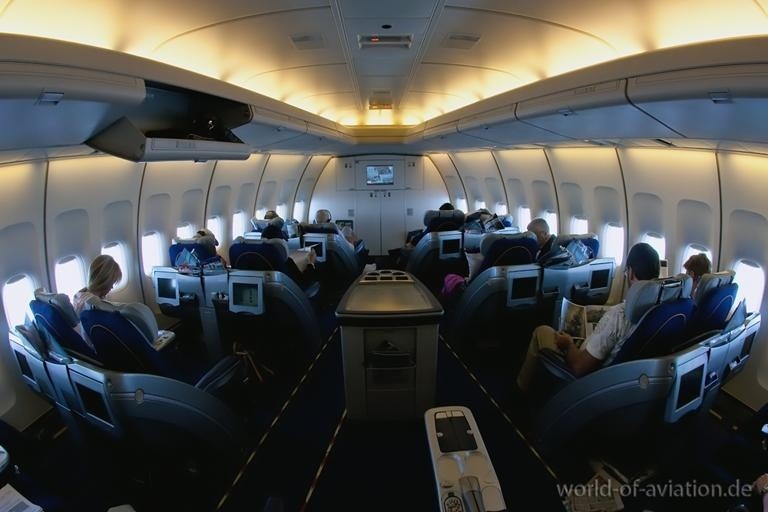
626, 243, 659, 279
261, 225, 288, 243
191, 229, 218, 246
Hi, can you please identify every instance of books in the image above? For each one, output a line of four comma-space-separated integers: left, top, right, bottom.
15, 313, 71, 366
709, 297, 762, 349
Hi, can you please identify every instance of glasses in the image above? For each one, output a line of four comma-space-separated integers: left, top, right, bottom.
622, 270, 630, 275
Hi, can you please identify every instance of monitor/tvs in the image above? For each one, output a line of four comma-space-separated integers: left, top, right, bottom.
511, 277, 536, 300
741, 331, 757, 363
233, 283, 259, 307
305, 241, 322, 257
443, 240, 460, 253
157, 278, 176, 299
677, 365, 703, 409
336, 219, 353, 233
366, 165, 394, 185
15, 350, 35, 384
77, 381, 113, 428
592, 269, 610, 291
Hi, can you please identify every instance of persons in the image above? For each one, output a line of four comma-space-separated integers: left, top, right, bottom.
71, 208, 369, 361
398, 201, 711, 405
